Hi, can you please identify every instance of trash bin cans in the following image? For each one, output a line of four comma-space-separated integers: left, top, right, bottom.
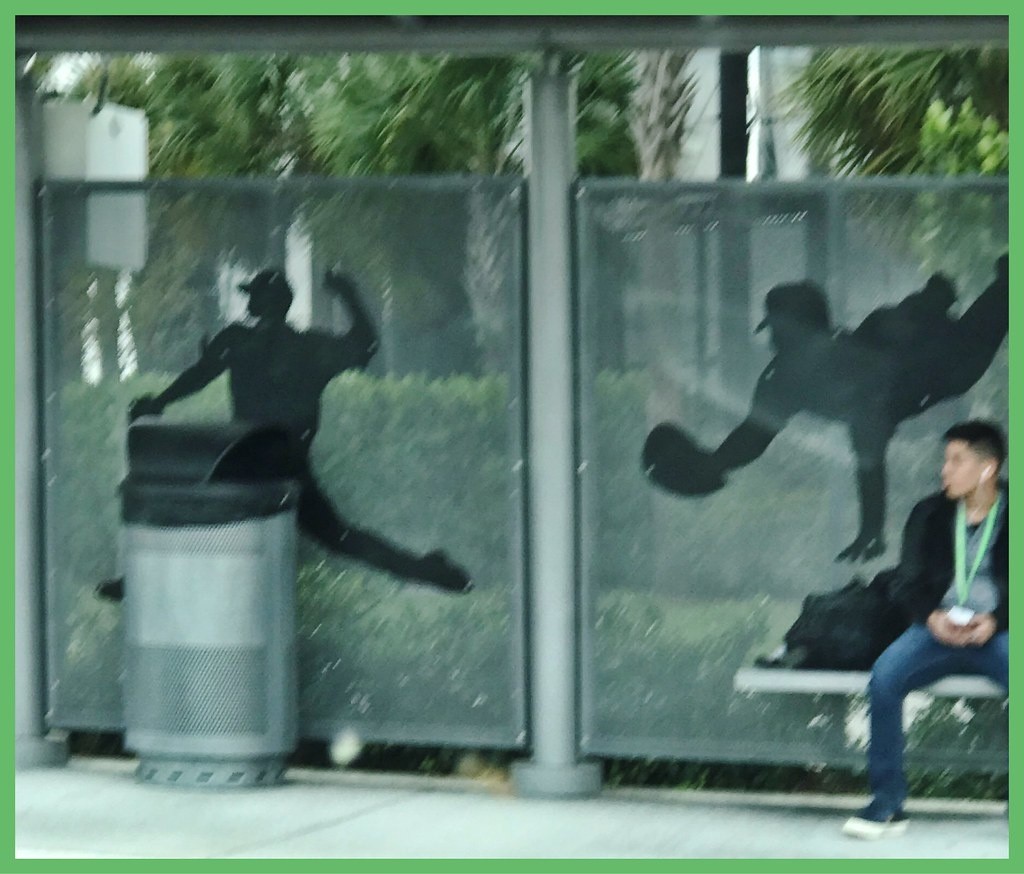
117, 423, 304, 794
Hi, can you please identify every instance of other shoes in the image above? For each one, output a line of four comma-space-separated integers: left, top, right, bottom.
843, 798, 910, 836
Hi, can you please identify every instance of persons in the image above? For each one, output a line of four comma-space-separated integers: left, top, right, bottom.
847, 419, 1009, 839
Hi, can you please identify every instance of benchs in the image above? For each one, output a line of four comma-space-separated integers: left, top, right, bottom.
734, 667, 1008, 700
